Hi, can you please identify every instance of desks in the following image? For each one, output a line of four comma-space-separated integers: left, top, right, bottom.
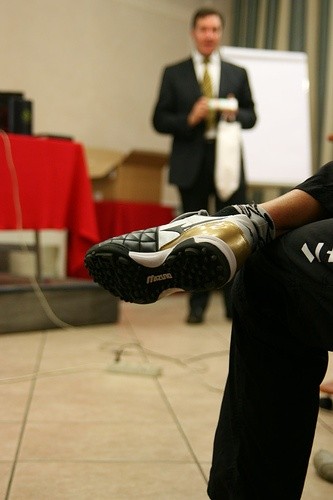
1, 135, 176, 281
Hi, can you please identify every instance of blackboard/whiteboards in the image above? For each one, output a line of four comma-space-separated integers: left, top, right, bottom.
216, 46, 315, 188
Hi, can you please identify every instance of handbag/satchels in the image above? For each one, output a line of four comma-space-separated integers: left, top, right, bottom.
213, 107, 244, 201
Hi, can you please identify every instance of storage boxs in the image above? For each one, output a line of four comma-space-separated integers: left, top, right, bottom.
85, 148, 170, 202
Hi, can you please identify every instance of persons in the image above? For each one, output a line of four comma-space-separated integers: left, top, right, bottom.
82, 162, 333, 500
151, 8, 256, 324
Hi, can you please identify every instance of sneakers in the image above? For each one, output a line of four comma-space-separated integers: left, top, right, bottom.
83, 210, 261, 305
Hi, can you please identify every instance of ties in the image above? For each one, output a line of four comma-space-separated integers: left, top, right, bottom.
201, 60, 215, 131
